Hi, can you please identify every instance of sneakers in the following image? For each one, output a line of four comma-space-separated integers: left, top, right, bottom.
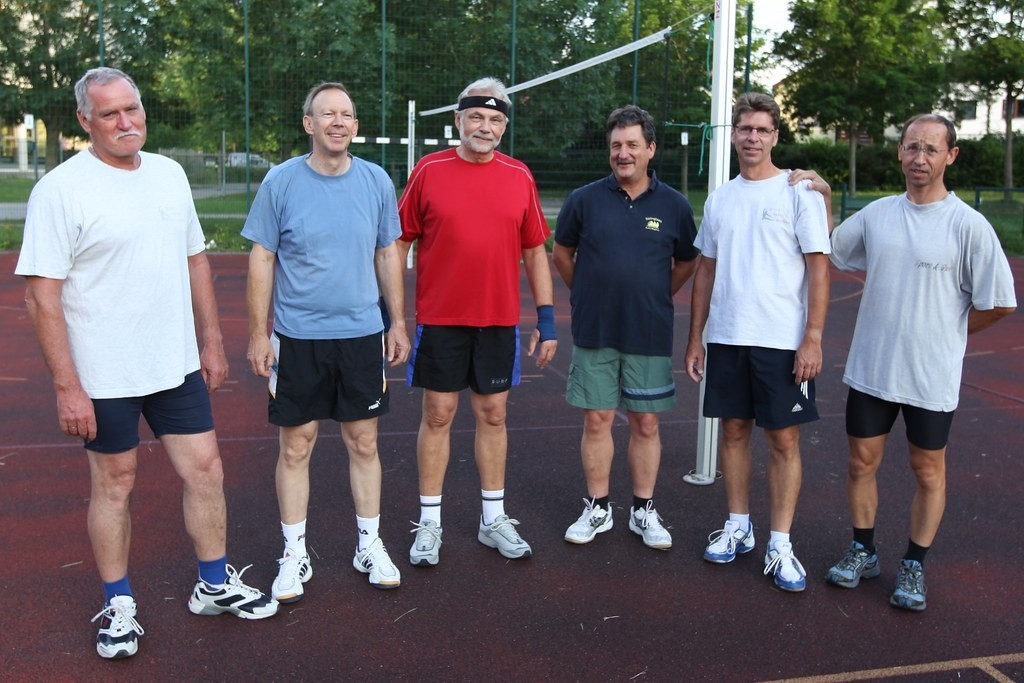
187, 563, 280, 619
565, 495, 614, 543
90, 595, 143, 658
630, 503, 673, 548
409, 518, 443, 564
272, 548, 314, 603
891, 560, 927, 611
828, 540, 881, 589
762, 537, 807, 592
353, 540, 401, 588
478, 514, 532, 560
703, 518, 756, 562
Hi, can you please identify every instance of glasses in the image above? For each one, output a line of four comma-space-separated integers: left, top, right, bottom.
899, 141, 949, 157
735, 126, 776, 138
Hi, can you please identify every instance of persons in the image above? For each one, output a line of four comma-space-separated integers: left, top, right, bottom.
379, 77, 557, 566
238, 81, 412, 603
784, 114, 1018, 611
683, 93, 830, 592
555, 104, 701, 550
13, 67, 281, 657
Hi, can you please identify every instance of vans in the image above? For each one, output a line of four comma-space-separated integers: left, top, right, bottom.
228, 152, 277, 169
